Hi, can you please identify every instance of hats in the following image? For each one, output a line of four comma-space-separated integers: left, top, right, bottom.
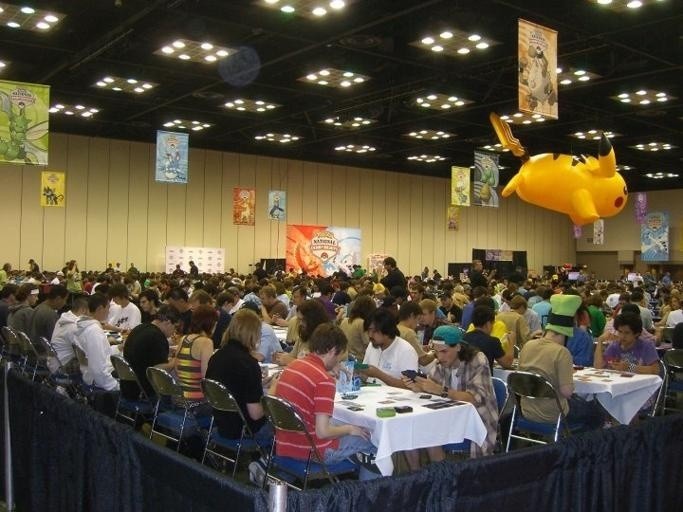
372, 282, 385, 294
437, 291, 451, 297
431, 325, 470, 346
545, 293, 583, 338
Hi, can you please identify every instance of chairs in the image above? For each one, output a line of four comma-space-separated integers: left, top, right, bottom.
200, 378, 275, 480
110, 354, 173, 432
1, 315, 683, 417
505, 370, 573, 454
442, 376, 510, 459
145, 367, 217, 453
259, 394, 360, 492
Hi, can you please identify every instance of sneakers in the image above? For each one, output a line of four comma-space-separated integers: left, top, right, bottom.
248, 456, 269, 486
